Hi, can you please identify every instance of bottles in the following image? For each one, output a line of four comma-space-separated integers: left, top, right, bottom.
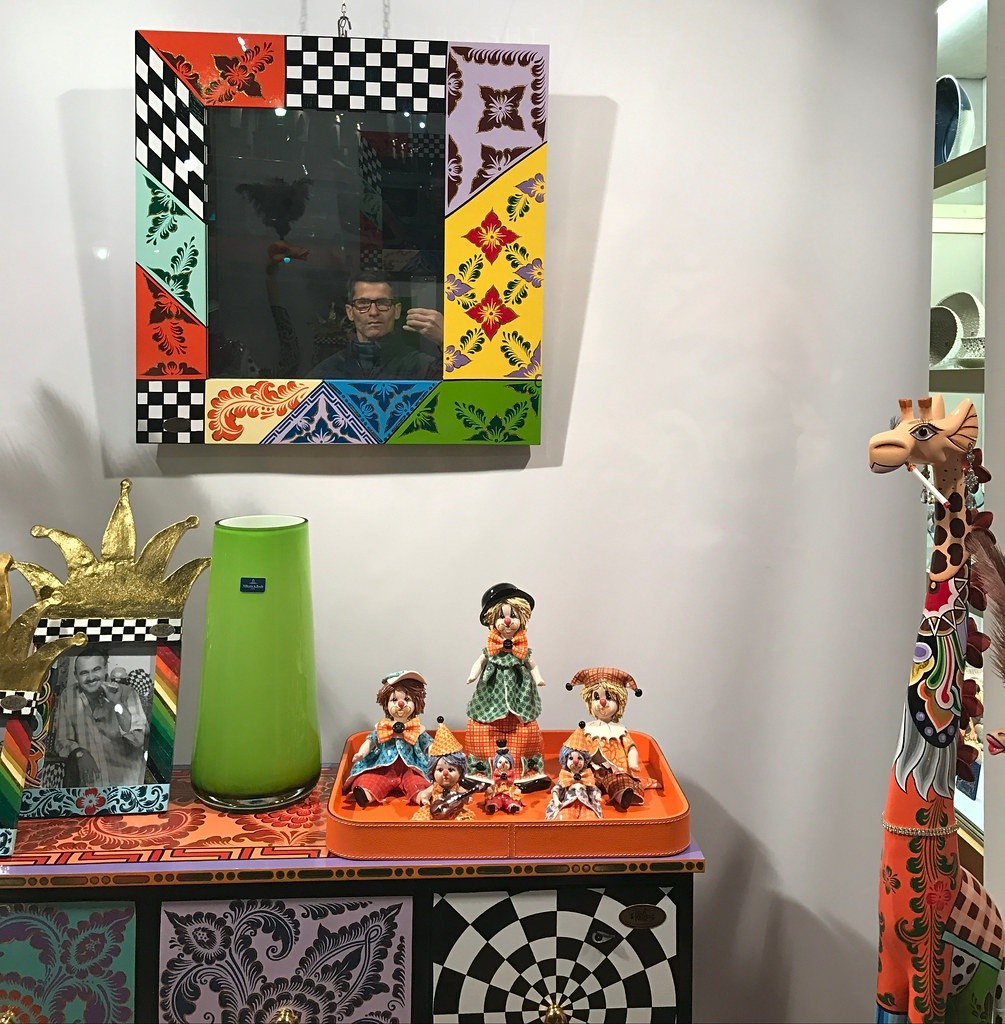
187, 513, 321, 812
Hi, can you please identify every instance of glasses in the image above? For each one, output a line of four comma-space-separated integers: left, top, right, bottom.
350, 297, 395, 314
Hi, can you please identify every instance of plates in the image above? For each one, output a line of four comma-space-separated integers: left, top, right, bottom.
957, 354, 989, 368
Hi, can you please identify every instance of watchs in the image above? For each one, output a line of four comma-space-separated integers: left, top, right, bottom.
75, 748, 84, 761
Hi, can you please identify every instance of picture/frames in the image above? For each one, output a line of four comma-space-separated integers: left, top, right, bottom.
8, 478, 210, 817
0, 550, 89, 856
135, 30, 551, 445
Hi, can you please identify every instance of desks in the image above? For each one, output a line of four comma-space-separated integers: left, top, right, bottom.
0, 763, 706, 1024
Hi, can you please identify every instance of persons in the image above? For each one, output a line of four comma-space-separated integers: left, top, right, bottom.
342, 670, 436, 808
207, 110, 366, 379
483, 739, 527, 814
302, 268, 444, 381
411, 715, 475, 822
465, 583, 546, 780
52, 649, 150, 788
559, 667, 644, 809
546, 721, 603, 820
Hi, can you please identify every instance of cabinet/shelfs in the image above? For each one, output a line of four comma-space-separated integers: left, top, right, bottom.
928, 0, 1005, 909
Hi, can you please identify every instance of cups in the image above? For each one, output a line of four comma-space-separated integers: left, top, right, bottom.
960, 336, 986, 357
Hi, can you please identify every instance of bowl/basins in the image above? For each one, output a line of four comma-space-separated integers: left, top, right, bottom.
934, 291, 986, 365
929, 306, 964, 369
934, 75, 974, 168
